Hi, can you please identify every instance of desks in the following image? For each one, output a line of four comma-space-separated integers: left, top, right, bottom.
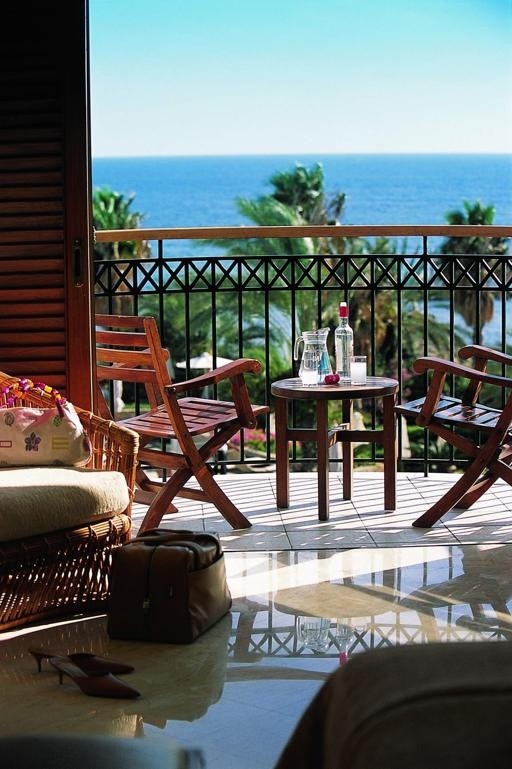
271, 377, 399, 521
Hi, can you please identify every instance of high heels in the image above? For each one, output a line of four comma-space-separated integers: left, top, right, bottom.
28, 647, 137, 677
45, 658, 142, 704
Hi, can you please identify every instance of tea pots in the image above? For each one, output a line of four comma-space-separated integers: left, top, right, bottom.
294, 327, 334, 385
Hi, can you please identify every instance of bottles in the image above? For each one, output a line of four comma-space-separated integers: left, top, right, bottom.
334, 301, 354, 381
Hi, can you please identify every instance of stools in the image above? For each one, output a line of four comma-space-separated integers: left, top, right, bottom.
2, 734, 204, 769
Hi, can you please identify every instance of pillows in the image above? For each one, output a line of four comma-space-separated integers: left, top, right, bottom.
0, 403, 93, 468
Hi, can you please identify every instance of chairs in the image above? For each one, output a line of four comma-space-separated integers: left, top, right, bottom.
393, 344, 512, 528
0, 370, 140, 634
95, 312, 271, 537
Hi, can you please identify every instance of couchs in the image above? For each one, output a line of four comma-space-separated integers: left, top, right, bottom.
274, 639, 512, 767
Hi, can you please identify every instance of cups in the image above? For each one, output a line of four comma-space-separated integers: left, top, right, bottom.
349, 355, 367, 386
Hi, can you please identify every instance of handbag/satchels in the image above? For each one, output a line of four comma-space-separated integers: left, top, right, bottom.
104, 529, 235, 643
1, 378, 96, 469
